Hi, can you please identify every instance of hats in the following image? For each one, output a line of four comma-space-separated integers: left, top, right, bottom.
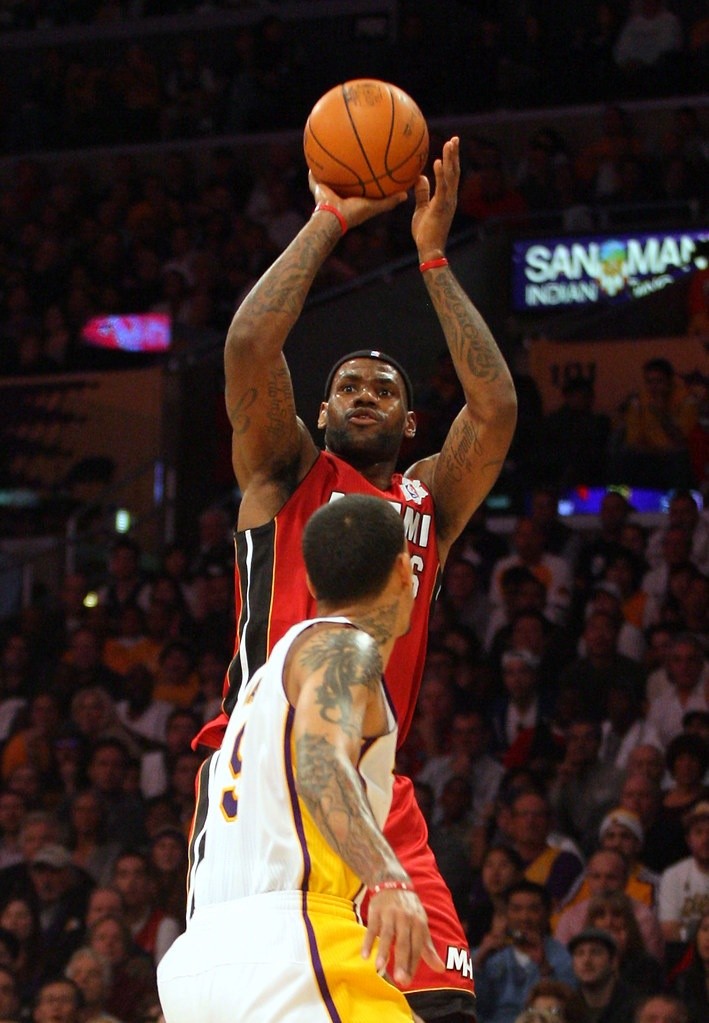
324, 350, 414, 411
565, 929, 617, 954
31, 842, 77, 869
598, 807, 646, 842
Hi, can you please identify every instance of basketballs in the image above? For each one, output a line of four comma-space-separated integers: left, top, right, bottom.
303, 78, 429, 201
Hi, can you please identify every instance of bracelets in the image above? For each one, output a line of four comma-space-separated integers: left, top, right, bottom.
314, 205, 347, 236
369, 882, 414, 894
419, 258, 448, 272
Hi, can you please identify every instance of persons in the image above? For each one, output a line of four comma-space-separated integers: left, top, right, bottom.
0, 0, 709, 380
396, 345, 709, 1023
193, 137, 519, 1023
0, 443, 240, 1023
156, 494, 444, 1023
